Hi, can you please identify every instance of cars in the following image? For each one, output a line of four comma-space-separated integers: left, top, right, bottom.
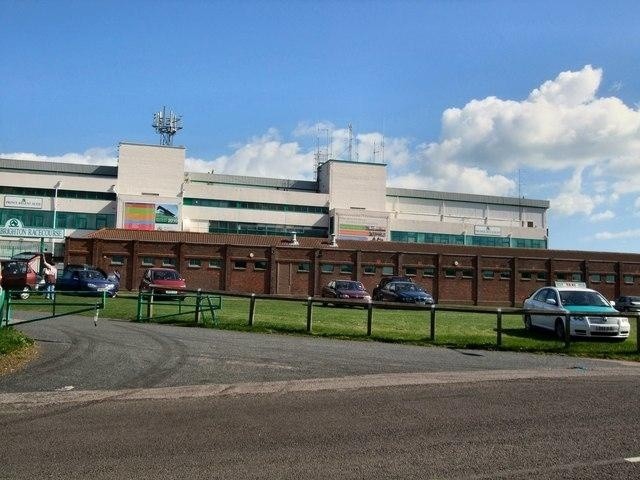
139, 268, 185, 301
523, 281, 630, 341
614, 296, 640, 312
61, 264, 121, 298
372, 276, 433, 304
322, 279, 370, 309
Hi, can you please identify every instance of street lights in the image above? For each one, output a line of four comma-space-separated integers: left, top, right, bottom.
52, 181, 62, 261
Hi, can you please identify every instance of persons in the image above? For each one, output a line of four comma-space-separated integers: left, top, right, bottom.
112, 268, 121, 292
42, 257, 58, 300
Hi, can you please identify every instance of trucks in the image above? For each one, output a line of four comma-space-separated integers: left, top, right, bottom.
1, 252, 52, 299
